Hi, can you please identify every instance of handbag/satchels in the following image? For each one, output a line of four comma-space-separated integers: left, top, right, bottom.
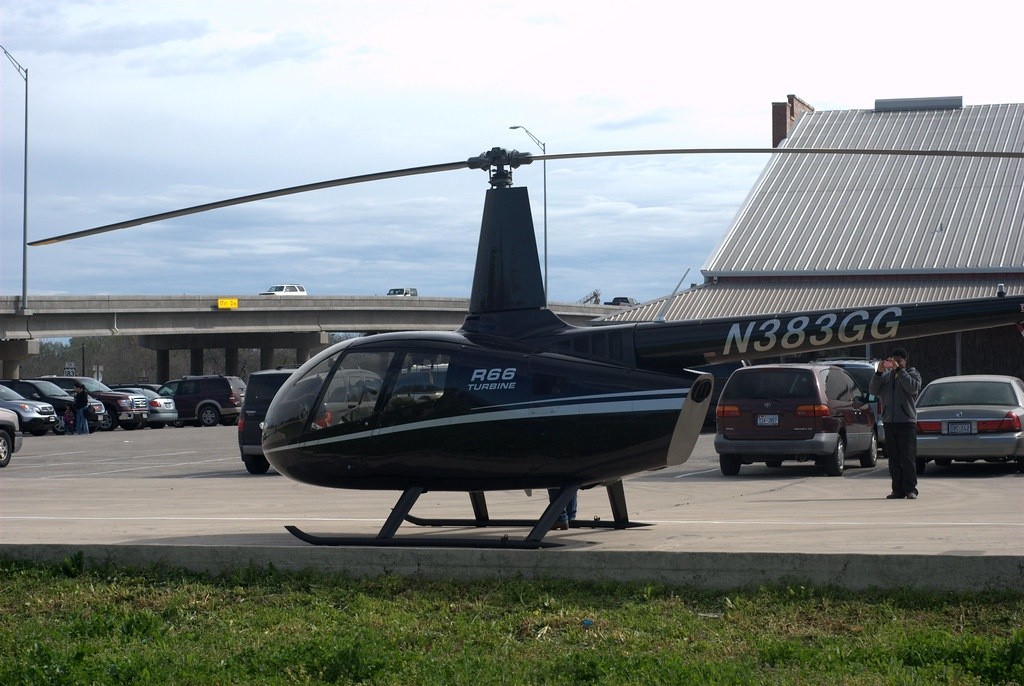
83, 405, 96, 418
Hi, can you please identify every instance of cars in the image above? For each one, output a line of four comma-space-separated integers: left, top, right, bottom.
0, 384, 59, 436
0, 407, 25, 467
108, 383, 174, 396
393, 361, 450, 403
111, 387, 178, 430
915, 373, 1024, 476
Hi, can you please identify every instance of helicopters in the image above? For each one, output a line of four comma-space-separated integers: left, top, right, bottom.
23, 145, 1024, 555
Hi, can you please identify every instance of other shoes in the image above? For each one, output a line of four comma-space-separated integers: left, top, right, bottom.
551, 518, 569, 530
887, 491, 906, 499
908, 492, 917, 499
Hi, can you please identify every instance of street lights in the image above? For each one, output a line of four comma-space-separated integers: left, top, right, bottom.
509, 126, 548, 306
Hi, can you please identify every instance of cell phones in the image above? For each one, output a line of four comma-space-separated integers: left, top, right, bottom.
884, 362, 894, 367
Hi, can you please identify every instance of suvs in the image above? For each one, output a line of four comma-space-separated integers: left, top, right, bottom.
385, 287, 418, 296
0, 379, 110, 435
155, 374, 249, 428
259, 284, 306, 296
34, 374, 150, 431
714, 362, 881, 476
788, 355, 889, 462
236, 365, 383, 476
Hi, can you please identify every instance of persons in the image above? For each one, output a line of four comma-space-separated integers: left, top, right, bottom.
869, 349, 922, 499
64, 382, 90, 435
